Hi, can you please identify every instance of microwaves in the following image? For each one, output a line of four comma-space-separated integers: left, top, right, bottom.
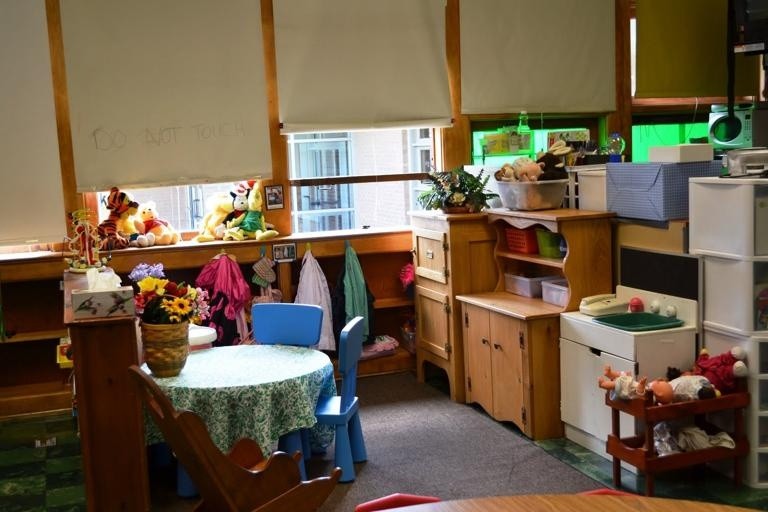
707, 109, 767, 153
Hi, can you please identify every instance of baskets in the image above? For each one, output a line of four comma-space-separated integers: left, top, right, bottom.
505, 229, 537, 253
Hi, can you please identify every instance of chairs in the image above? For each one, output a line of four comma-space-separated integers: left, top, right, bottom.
129, 363, 344, 512
279, 315, 370, 482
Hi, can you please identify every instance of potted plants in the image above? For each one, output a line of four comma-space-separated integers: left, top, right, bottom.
420, 164, 488, 215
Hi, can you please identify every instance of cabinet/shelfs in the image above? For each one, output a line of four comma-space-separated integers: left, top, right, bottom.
0, 164, 418, 424
455, 205, 617, 441
695, 254, 767, 490
606, 388, 751, 490
406, 210, 498, 403
558, 285, 698, 479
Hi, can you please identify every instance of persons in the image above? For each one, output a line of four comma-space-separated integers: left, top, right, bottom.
275, 189, 282, 204
598, 346, 747, 406
273, 245, 295, 259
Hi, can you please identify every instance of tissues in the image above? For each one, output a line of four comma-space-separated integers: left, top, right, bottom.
72, 267, 136, 321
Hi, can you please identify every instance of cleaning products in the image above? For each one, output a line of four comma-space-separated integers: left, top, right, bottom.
516, 110, 535, 154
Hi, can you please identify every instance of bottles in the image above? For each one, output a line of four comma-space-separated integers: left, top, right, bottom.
517, 111, 533, 154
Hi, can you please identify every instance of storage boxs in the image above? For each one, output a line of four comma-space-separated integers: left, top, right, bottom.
575, 163, 767, 259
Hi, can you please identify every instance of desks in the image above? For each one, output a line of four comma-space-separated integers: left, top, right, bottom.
370, 492, 767, 512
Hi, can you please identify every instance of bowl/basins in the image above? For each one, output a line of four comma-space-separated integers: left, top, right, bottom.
497, 179, 568, 210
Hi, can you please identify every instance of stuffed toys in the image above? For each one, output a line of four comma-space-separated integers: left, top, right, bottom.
99, 187, 137, 252
495, 140, 575, 209
133, 203, 182, 244
200, 180, 278, 243
118, 217, 155, 247
65, 207, 113, 273
